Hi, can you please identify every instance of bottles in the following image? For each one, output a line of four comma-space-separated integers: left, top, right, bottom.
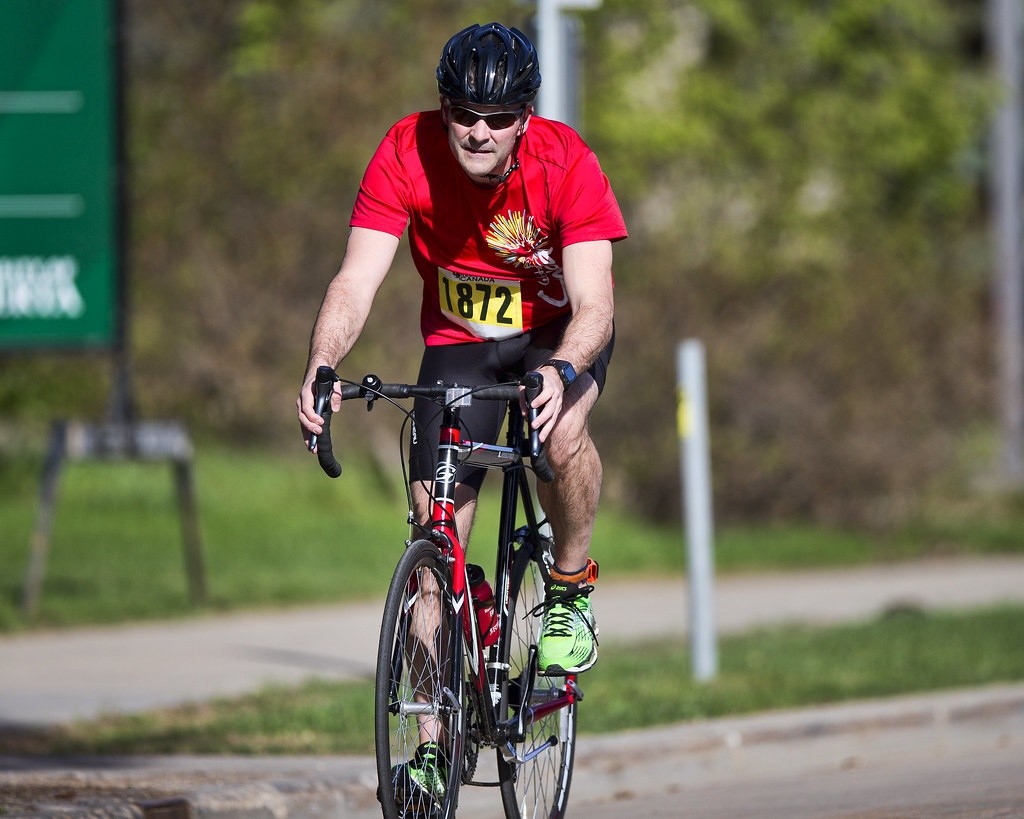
463, 563, 501, 648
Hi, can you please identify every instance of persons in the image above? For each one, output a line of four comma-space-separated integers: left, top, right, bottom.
295, 21, 628, 819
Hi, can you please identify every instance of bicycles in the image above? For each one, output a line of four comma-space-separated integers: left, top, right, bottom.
303, 364, 584, 819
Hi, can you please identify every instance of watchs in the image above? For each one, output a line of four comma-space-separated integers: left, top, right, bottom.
538, 359, 576, 392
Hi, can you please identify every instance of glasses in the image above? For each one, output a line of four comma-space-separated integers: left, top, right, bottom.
448, 104, 524, 130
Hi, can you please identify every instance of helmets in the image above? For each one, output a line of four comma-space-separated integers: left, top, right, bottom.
435, 22, 542, 107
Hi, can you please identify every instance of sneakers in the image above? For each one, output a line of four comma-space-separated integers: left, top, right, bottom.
522, 575, 600, 676
392, 741, 450, 802
